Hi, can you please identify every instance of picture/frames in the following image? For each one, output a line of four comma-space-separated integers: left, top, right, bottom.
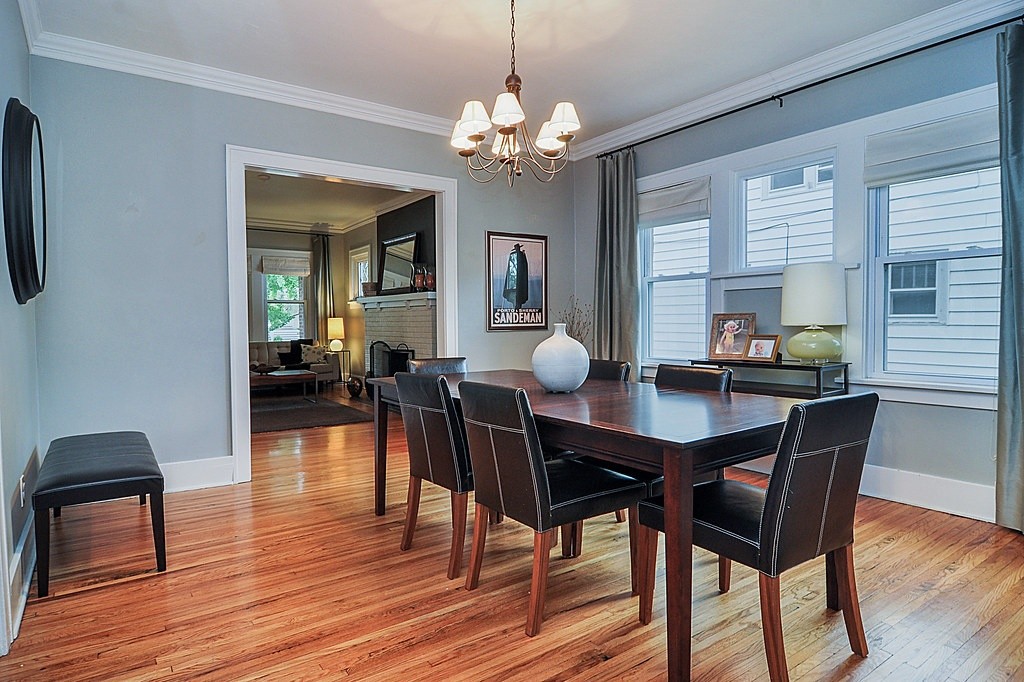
485, 229, 548, 331
741, 333, 783, 363
708, 313, 757, 357
2, 96, 47, 304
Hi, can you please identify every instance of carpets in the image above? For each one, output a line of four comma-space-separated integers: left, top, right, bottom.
249, 394, 375, 433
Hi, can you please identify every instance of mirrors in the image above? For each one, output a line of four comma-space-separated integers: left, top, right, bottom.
374, 231, 421, 295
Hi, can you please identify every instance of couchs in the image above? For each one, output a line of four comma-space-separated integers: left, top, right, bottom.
250, 341, 339, 394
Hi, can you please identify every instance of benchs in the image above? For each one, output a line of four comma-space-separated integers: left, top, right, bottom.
30, 430, 168, 597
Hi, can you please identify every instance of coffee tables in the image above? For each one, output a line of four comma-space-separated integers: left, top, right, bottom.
249, 369, 318, 404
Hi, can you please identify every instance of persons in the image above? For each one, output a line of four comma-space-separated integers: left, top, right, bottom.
720, 321, 742, 353
755, 341, 764, 357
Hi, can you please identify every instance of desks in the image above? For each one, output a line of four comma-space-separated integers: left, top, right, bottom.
360, 368, 815, 682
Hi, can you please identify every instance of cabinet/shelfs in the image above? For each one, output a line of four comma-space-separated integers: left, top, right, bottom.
689, 358, 852, 401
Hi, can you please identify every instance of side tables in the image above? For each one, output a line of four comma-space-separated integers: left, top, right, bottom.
328, 350, 351, 387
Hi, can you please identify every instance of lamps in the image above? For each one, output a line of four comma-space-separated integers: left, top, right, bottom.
449, 0, 581, 189
780, 262, 847, 363
327, 317, 345, 352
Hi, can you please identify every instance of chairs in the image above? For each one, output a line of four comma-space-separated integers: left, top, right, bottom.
638, 391, 880, 682
585, 358, 632, 522
392, 373, 572, 586
408, 355, 500, 524
456, 378, 650, 632
652, 363, 734, 482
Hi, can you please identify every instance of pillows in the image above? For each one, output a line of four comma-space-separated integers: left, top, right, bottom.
300, 343, 327, 364
277, 351, 302, 366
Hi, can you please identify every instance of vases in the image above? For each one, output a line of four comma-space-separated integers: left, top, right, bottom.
532, 322, 590, 393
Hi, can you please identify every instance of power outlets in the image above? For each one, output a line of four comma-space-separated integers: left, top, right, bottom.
19, 475, 27, 508
